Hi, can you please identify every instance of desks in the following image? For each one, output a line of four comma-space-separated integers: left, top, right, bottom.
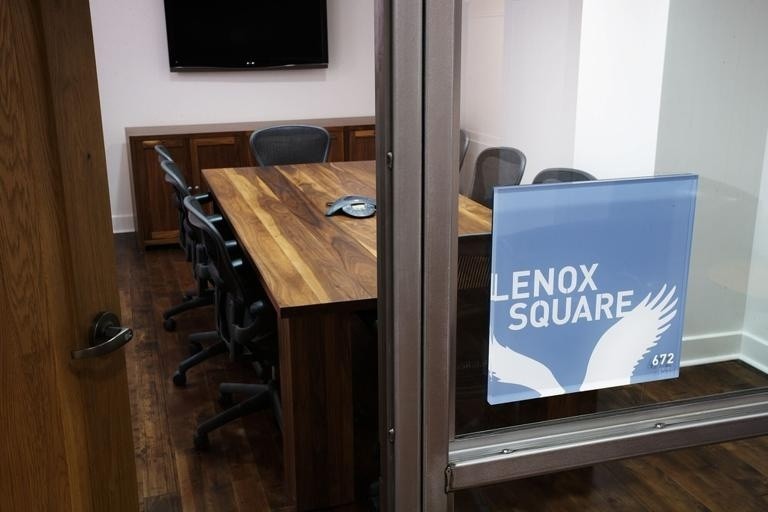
200, 160, 492, 510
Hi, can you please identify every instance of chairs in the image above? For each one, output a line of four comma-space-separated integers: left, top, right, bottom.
461, 128, 469, 166
249, 125, 330, 167
471, 148, 526, 210
155, 144, 291, 452
531, 167, 595, 186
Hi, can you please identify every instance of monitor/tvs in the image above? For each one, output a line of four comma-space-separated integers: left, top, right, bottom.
164, 0, 329, 73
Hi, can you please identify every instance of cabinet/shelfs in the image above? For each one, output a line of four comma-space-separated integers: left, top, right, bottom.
124, 126, 241, 251
